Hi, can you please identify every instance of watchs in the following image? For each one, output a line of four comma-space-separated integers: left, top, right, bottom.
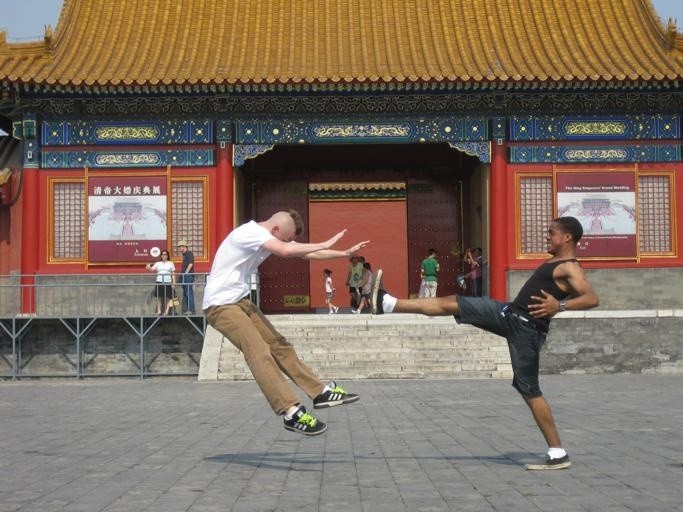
559, 299, 565, 312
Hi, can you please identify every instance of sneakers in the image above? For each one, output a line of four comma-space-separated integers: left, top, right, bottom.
524, 454, 570, 470
369, 269, 388, 315
351, 309, 360, 314
313, 381, 359, 409
282, 405, 327, 436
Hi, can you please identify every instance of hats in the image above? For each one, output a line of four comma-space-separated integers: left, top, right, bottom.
176, 241, 188, 247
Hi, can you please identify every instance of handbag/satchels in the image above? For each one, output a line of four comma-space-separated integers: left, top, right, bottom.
169, 298, 179, 307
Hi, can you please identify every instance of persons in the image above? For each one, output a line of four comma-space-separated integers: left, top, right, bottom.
145, 249, 177, 316
321, 268, 340, 315
198, 207, 372, 437
367, 214, 600, 472
345, 247, 482, 315
176, 239, 196, 315
